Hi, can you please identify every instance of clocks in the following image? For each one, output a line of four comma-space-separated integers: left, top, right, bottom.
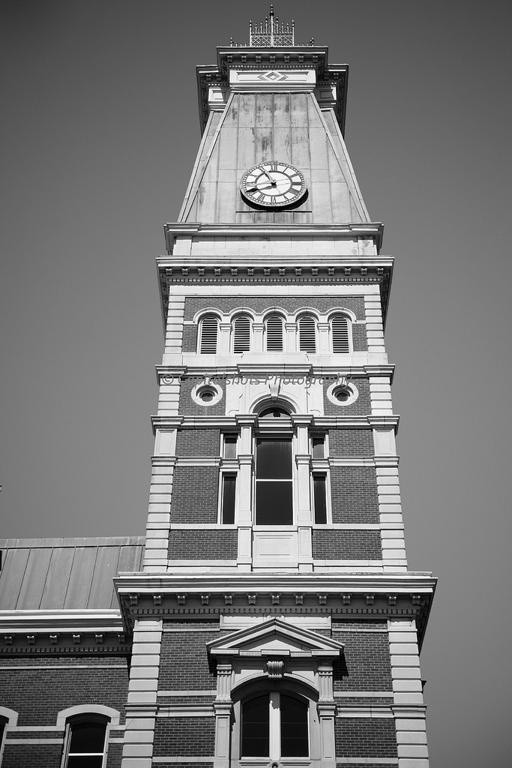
240, 161, 307, 208
